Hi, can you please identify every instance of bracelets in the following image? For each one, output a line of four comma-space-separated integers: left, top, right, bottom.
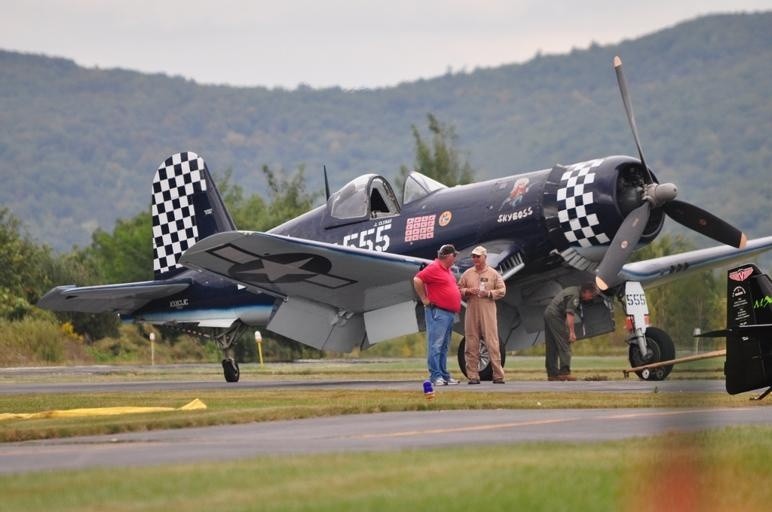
487, 290, 492, 299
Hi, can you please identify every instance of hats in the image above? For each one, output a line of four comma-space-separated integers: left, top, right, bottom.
471, 246, 488, 256
440, 245, 459, 254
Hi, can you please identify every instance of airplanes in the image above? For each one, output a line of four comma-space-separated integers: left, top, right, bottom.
37, 56, 772, 381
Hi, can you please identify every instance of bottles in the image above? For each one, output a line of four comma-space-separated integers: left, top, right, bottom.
478, 282, 485, 297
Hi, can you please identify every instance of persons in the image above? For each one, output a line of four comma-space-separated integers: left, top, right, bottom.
412, 243, 463, 390
457, 246, 510, 386
542, 281, 601, 382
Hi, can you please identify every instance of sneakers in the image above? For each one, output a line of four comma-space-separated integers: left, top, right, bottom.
493, 378, 505, 383
548, 374, 576, 381
432, 376, 458, 385
468, 378, 481, 384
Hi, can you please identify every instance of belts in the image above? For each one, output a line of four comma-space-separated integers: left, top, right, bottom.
429, 304, 455, 313
